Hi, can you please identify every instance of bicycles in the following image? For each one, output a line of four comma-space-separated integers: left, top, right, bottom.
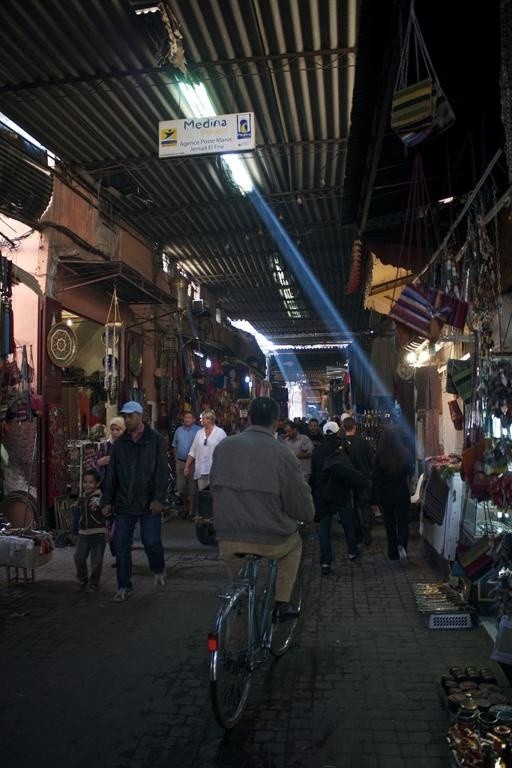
165, 452, 185, 519
207, 519, 303, 728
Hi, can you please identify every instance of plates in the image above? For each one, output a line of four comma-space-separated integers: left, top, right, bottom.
47, 322, 78, 368
103, 354, 118, 370
101, 330, 120, 347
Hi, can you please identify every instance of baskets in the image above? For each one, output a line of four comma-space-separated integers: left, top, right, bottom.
425, 613, 472, 629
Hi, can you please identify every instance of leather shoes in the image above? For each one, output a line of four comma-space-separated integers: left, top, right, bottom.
272, 602, 302, 624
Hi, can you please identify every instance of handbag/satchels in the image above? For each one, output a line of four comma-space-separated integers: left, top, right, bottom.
408, 477, 418, 496
0, 361, 45, 421
457, 535, 497, 576
336, 446, 370, 490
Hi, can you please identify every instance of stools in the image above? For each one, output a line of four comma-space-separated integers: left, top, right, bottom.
4, 565, 36, 584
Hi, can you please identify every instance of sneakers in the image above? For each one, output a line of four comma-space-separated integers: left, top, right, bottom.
321, 535, 408, 575
71, 566, 168, 603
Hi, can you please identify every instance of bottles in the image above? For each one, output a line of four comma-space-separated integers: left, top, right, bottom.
361, 409, 394, 450
438, 690, 512, 753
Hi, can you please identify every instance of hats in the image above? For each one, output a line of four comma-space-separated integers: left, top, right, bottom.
322, 421, 340, 435
119, 401, 144, 415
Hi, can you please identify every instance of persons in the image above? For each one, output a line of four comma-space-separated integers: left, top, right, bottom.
72, 402, 250, 602
209, 396, 315, 625
276, 409, 411, 573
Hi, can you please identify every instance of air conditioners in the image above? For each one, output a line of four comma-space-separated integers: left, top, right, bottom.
192, 299, 210, 311
